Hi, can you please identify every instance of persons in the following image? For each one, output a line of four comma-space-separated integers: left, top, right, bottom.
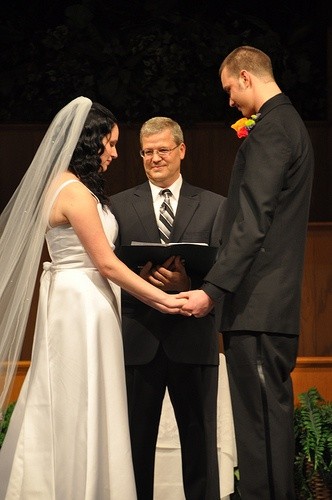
0, 96, 189, 500
175, 45, 315, 500
105, 116, 229, 500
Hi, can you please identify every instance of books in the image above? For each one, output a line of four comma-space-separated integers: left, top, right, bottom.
119, 240, 218, 294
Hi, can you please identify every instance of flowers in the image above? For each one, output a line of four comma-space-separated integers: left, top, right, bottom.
231, 113, 261, 139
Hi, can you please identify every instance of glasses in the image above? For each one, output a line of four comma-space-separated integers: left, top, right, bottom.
140, 144, 179, 157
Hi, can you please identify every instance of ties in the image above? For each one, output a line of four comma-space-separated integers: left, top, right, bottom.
158, 190, 174, 244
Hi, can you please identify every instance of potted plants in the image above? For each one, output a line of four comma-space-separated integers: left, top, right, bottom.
293, 385, 332, 500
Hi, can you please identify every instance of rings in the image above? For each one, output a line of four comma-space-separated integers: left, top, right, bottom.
188, 313, 192, 316
